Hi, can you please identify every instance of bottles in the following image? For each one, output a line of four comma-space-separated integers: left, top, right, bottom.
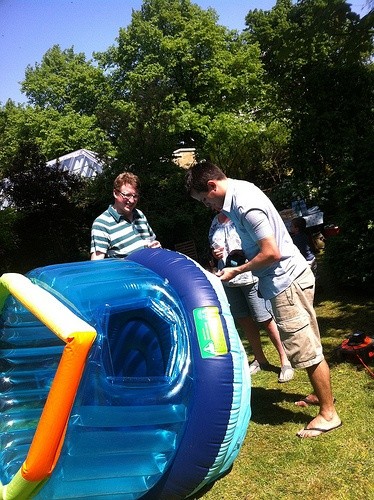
299, 199, 309, 217
291, 201, 299, 217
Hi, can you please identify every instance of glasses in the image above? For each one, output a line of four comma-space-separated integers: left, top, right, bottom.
117, 190, 140, 199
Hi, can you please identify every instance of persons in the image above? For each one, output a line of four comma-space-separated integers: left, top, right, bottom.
186, 162, 343, 437
90, 172, 162, 260
290, 217, 322, 293
209, 203, 295, 383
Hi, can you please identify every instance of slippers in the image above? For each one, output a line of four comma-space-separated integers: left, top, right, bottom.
279, 365, 294, 382
296, 420, 343, 439
295, 398, 336, 406
249, 360, 269, 375
